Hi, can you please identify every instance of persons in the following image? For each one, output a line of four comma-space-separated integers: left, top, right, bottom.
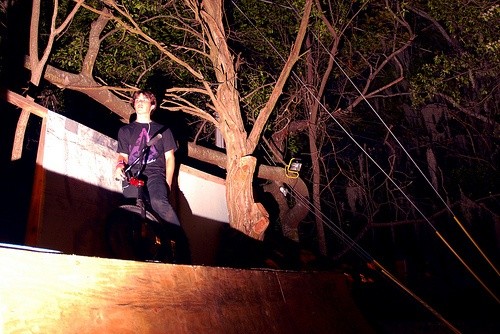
117, 89, 192, 265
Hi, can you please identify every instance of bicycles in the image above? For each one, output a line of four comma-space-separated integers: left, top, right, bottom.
95, 133, 177, 264
